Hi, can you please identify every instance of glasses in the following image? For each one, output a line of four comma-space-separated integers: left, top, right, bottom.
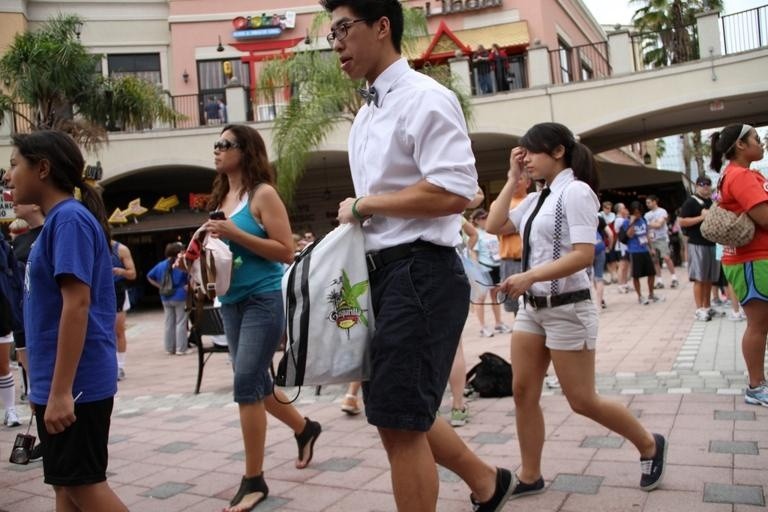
468, 278, 508, 306
212, 138, 241, 152
327, 17, 369, 48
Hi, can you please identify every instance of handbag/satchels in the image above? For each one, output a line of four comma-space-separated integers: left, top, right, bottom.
700, 173, 756, 247
270, 218, 377, 404
618, 226, 629, 244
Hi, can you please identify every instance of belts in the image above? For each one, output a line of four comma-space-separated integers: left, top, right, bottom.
527, 287, 592, 310
366, 237, 456, 274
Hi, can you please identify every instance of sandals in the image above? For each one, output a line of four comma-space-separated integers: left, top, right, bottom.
293, 416, 322, 470
222, 470, 270, 511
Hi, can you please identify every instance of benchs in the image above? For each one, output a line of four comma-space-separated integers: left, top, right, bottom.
184, 303, 233, 395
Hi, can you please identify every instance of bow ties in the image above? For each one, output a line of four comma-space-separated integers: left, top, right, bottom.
355, 85, 380, 107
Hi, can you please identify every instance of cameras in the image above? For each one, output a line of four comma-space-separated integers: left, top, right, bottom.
9, 433, 36, 465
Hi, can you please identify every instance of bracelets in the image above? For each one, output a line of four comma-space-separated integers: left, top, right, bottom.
350, 194, 375, 220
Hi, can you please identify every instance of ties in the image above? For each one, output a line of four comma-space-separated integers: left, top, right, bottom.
521, 186, 551, 311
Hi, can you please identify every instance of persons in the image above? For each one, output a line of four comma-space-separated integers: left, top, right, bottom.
169, 122, 323, 512
482, 120, 671, 503
341, 380, 361, 415
3, 129, 135, 512
711, 122, 768, 406
216, 97, 227, 124
464, 164, 532, 337
321, 1, 518, 511
436, 339, 472, 425
1, 203, 48, 462
472, 43, 494, 95
292, 233, 320, 251
110, 239, 137, 380
203, 95, 222, 127
147, 242, 189, 356
489, 43, 512, 94
585, 175, 747, 321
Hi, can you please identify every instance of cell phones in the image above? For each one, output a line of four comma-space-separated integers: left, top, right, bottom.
209, 211, 226, 234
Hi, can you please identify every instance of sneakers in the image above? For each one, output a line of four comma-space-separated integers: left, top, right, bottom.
470, 466, 519, 512
176, 348, 193, 355
745, 384, 768, 407
480, 329, 494, 337
636, 433, 671, 492
451, 405, 471, 426
3, 408, 22, 427
509, 474, 547, 499
341, 394, 362, 415
694, 294, 747, 321
619, 280, 679, 305
117, 368, 127, 381
495, 324, 512, 333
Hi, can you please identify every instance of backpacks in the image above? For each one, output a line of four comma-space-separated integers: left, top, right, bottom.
178, 221, 234, 319
158, 260, 174, 296
463, 350, 512, 400
